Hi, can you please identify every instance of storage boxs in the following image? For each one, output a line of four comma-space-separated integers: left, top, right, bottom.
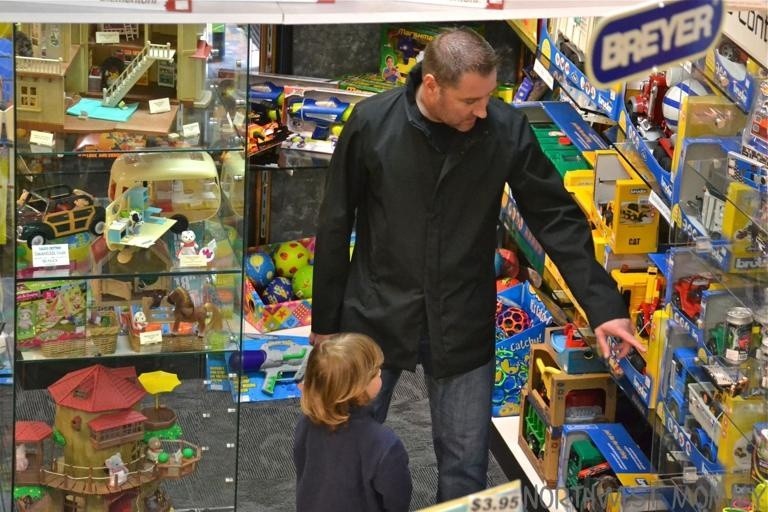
107, 151, 223, 226
15, 277, 87, 349
204, 233, 357, 403
340, 24, 461, 94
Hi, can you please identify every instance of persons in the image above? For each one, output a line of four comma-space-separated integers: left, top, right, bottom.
308, 23, 648, 506
291, 330, 413, 510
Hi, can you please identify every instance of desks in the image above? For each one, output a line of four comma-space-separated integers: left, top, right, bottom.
248, 149, 337, 245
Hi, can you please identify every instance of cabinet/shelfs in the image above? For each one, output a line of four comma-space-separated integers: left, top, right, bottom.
655, 151, 767, 511
5, 18, 247, 512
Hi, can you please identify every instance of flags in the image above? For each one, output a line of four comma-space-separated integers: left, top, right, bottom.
1, 23, 226, 512
